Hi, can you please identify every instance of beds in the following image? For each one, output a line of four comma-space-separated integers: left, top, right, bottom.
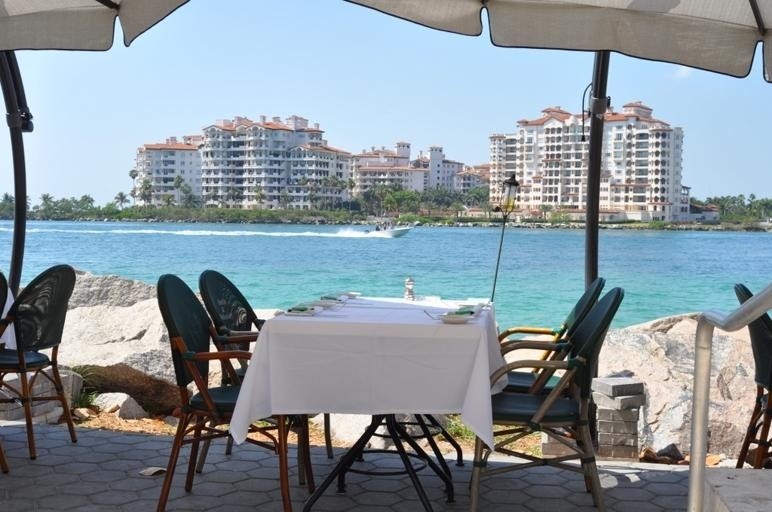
347, 0, 770, 446
0, 1, 194, 293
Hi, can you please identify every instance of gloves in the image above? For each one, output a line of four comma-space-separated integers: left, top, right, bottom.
363, 226, 411, 240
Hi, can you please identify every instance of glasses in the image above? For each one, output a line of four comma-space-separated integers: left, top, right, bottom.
490, 172, 519, 302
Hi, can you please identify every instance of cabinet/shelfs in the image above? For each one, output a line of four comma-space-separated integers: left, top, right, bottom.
404, 274, 416, 302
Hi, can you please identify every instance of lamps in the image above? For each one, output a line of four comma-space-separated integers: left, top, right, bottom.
465, 286, 630, 512
192, 269, 338, 487
464, 275, 609, 487
153, 273, 315, 512
1, 263, 79, 479
730, 281, 771, 467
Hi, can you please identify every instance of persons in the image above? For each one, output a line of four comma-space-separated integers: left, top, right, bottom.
0, 273, 19, 354
376, 220, 390, 231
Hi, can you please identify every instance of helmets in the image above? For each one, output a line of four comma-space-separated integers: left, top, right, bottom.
344, 291, 360, 298
440, 315, 469, 324
